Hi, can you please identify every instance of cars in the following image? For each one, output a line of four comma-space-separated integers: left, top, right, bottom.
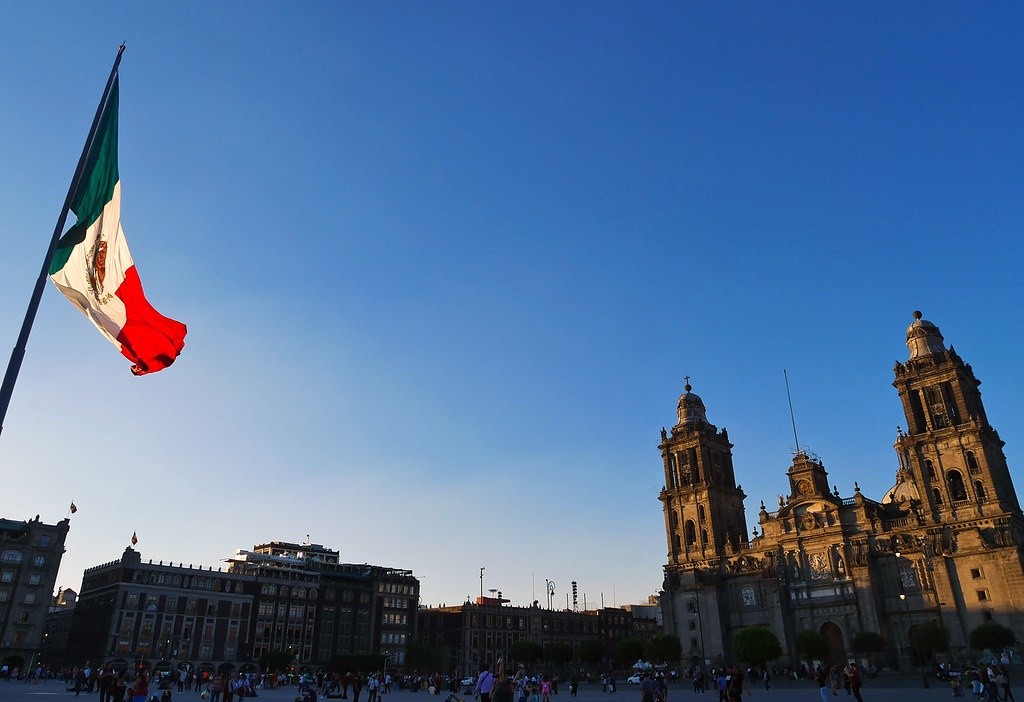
460, 677, 477, 686
627, 672, 646, 685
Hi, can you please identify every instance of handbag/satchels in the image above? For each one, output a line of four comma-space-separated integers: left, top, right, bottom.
238, 687, 245, 696
201, 689, 210, 700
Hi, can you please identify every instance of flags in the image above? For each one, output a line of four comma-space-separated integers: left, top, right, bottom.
131, 532, 138, 545
48, 68, 188, 377
70, 503, 77, 514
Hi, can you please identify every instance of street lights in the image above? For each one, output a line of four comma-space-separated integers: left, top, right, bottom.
384, 654, 406, 676
689, 559, 710, 691
480, 567, 485, 605
889, 534, 930, 689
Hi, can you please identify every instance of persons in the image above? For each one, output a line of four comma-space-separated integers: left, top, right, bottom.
639, 667, 691, 702
475, 663, 578, 702
175, 665, 288, 702
691, 663, 771, 702
398, 668, 465, 702
783, 660, 863, 702
936, 649, 1024, 702
66, 662, 172, 702
600, 671, 617, 694
0, 661, 69, 684
295, 670, 392, 702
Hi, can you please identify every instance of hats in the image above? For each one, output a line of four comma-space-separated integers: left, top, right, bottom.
492, 672, 499, 678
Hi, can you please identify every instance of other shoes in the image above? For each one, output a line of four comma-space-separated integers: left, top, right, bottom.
847, 692, 851, 695
555, 692, 558, 695
320, 696, 326, 699
832, 693, 837, 696
